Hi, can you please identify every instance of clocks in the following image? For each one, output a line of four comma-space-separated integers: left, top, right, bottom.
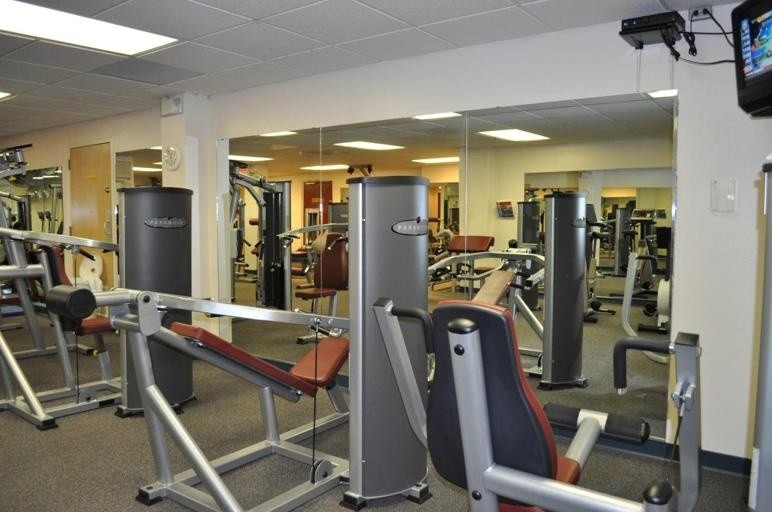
163, 144, 180, 170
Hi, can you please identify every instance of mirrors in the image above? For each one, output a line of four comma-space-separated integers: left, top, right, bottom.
0, 166, 63, 297
521, 167, 673, 303
213, 88, 679, 446
114, 146, 162, 275
427, 183, 460, 234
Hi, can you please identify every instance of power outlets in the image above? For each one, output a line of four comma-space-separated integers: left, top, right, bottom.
689, 6, 712, 21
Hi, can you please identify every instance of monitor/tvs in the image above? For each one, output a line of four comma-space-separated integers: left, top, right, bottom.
730, 1, 772, 120
